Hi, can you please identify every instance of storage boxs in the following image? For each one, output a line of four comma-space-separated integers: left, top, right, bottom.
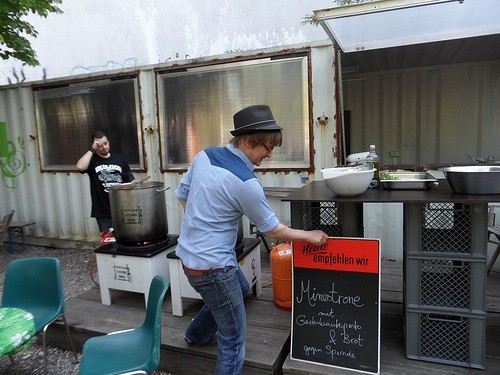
290, 201, 488, 369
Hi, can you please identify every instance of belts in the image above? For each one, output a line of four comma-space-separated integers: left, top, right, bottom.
181, 263, 221, 274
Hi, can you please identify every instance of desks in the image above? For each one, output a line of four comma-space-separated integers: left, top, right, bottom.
0, 307, 37, 359
0, 221, 36, 255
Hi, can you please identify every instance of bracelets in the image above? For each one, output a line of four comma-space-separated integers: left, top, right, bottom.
88, 146, 95, 153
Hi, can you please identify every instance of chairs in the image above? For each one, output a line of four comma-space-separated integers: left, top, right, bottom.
3, 257, 79, 375
77, 275, 168, 375
0, 209, 16, 243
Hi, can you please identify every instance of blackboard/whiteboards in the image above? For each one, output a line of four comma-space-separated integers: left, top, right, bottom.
290, 237, 381, 375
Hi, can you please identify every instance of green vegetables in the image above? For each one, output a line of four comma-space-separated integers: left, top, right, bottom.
382, 173, 400, 180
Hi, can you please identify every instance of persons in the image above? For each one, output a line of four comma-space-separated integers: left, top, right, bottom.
175, 104, 330, 375
76, 131, 135, 232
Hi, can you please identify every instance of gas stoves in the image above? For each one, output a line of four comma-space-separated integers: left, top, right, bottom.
112, 236, 170, 250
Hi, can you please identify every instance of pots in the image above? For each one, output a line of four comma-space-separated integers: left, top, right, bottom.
105, 182, 171, 244
234, 215, 244, 248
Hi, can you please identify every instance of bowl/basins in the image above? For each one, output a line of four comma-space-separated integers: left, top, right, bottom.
440, 166, 500, 194
320, 165, 377, 196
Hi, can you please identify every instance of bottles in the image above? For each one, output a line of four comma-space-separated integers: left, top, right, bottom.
366, 144, 381, 188
347, 150, 370, 163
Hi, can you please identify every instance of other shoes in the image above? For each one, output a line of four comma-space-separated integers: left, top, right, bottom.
184, 332, 218, 346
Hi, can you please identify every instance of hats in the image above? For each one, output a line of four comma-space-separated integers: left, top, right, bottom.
230, 104, 284, 137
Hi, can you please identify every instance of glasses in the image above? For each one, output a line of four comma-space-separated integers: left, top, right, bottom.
261, 143, 274, 153
